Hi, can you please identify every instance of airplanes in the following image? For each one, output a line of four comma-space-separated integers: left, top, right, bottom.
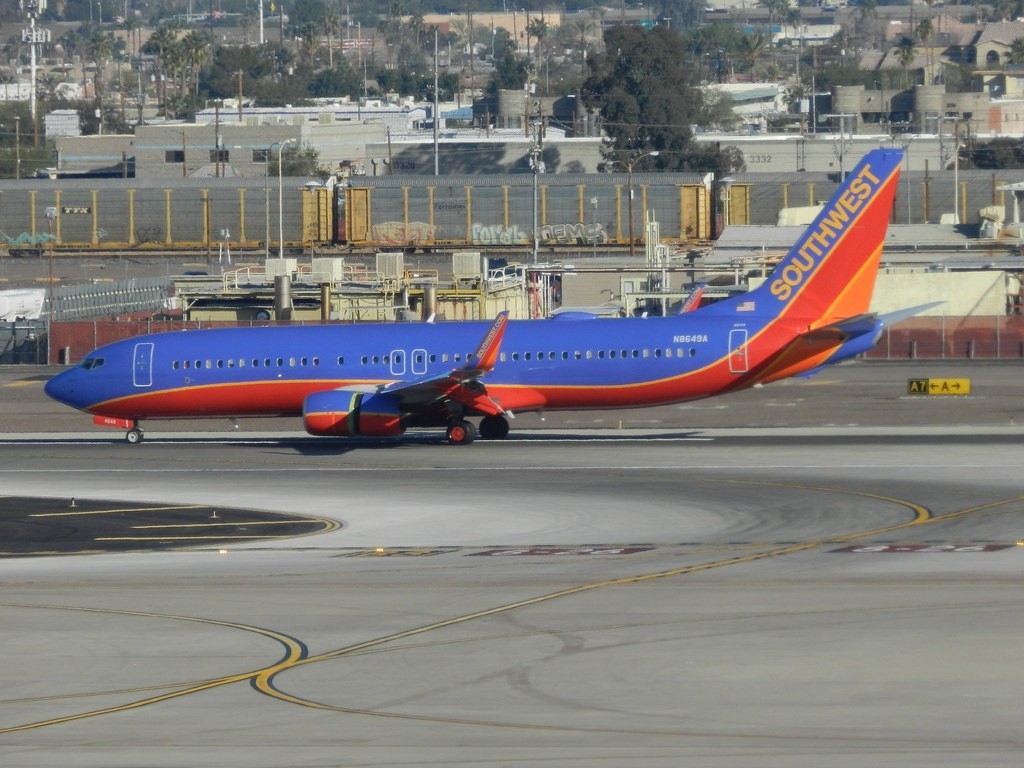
43, 143, 906, 447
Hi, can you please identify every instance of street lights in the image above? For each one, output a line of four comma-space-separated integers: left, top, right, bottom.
278, 138, 297, 258
14, 116, 20, 179
953, 143, 967, 226
606, 151, 660, 256
